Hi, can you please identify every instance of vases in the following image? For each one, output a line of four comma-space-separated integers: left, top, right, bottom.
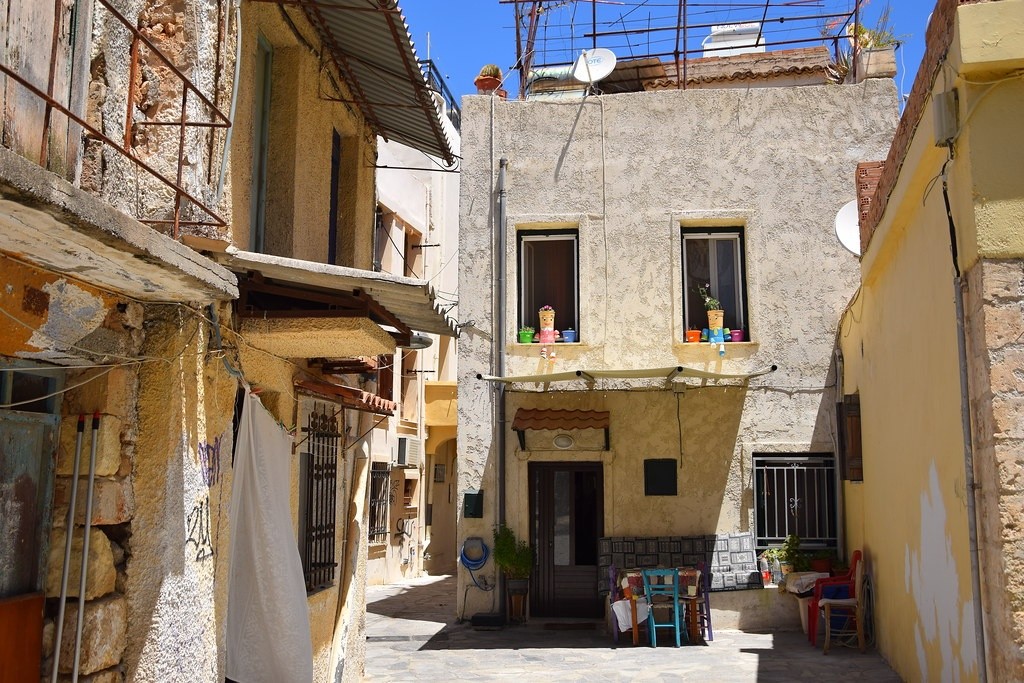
539, 311, 557, 343
706, 310, 725, 343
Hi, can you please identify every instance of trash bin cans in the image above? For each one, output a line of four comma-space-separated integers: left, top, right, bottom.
790, 573, 830, 635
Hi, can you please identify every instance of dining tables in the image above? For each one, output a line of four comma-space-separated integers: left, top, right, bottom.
624, 566, 701, 646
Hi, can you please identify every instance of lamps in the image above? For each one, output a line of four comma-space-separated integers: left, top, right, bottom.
553, 434, 575, 449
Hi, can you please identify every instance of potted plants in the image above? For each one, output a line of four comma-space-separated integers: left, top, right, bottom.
519, 325, 535, 343
757, 534, 850, 589
474, 65, 503, 95
492, 519, 537, 624
849, 24, 898, 82
686, 323, 702, 342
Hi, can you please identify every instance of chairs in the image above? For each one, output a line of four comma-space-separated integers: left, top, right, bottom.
609, 566, 713, 647
808, 550, 866, 656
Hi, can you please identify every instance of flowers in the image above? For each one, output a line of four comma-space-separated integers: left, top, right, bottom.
540, 305, 554, 312
691, 283, 720, 310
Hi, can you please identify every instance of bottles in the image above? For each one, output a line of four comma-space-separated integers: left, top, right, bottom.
760, 554, 782, 585
